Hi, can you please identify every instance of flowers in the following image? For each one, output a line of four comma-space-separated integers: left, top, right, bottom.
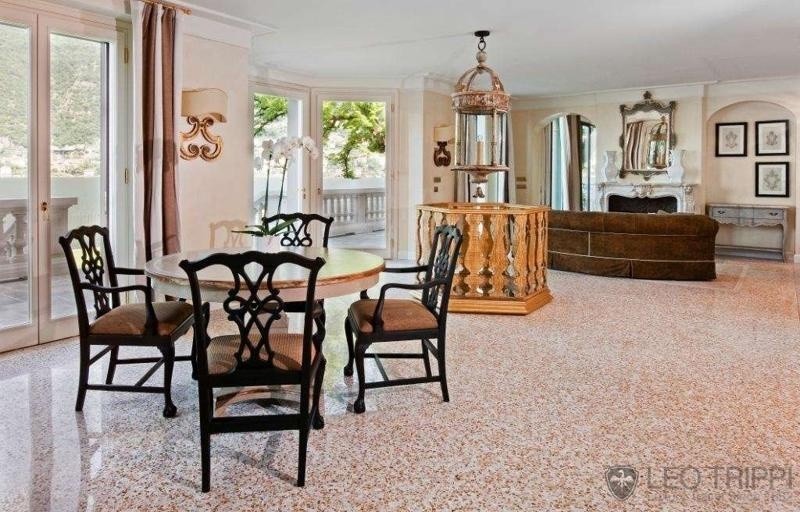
229, 135, 318, 237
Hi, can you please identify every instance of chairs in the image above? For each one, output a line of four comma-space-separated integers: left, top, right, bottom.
261, 212, 333, 247
57, 224, 197, 418
177, 251, 326, 493
344, 224, 463, 413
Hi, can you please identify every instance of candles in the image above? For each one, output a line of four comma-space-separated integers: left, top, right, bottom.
477, 141, 483, 165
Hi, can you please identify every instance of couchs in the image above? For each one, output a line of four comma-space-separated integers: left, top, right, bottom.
546, 210, 719, 282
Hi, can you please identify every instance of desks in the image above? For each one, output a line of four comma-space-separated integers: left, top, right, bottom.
708, 203, 789, 263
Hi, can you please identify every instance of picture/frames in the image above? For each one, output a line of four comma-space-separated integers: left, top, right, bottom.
754, 119, 789, 156
714, 122, 747, 157
755, 161, 789, 197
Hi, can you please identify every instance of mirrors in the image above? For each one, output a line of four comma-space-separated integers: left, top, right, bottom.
618, 92, 677, 181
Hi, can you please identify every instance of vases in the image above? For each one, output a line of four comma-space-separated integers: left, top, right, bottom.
602, 151, 619, 182
667, 150, 686, 183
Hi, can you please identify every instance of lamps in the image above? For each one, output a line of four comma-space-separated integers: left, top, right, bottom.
433, 126, 454, 167
450, 31, 512, 200
178, 88, 229, 161
646, 115, 667, 168
